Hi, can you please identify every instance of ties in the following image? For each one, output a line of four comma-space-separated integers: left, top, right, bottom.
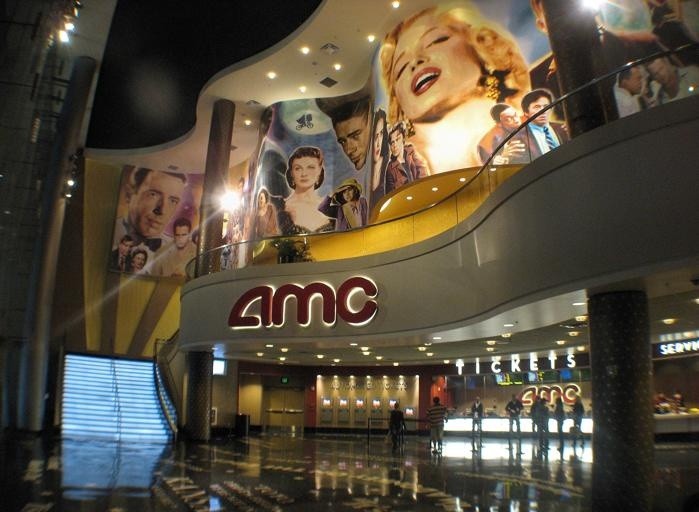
543, 127, 558, 150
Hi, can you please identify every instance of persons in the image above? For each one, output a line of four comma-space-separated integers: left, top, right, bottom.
505, 392, 523, 438
219, 0, 698, 239
471, 395, 483, 436
426, 396, 448, 454
654, 389, 685, 413
389, 402, 406, 450
555, 396, 565, 438
111, 168, 198, 279
530, 395, 550, 438
570, 395, 583, 428
